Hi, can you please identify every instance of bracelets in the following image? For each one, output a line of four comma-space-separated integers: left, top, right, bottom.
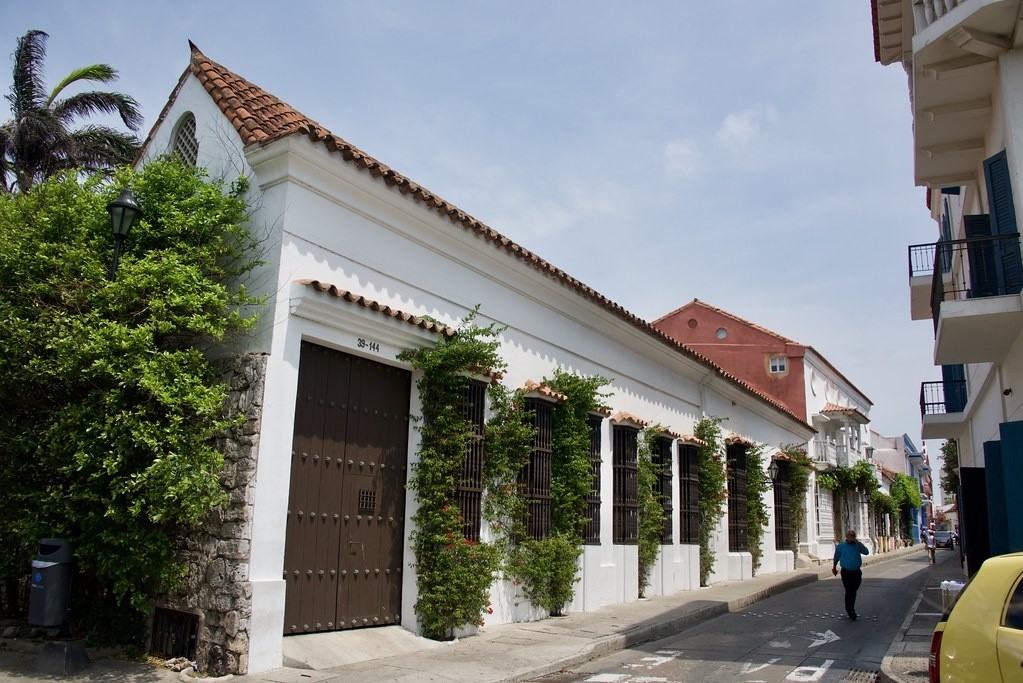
857, 541, 860, 544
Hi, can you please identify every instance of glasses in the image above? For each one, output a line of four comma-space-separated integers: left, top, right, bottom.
848, 534, 856, 538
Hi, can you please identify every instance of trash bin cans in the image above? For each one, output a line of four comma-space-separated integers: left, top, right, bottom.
28, 537, 78, 633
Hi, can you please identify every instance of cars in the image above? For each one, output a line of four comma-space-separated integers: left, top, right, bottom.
933, 531, 956, 549
929, 551, 1023, 683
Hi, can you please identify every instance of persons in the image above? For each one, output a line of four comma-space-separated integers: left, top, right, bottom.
949, 532, 953, 549
831, 529, 869, 620
955, 531, 959, 546
919, 530, 926, 543
925, 530, 936, 564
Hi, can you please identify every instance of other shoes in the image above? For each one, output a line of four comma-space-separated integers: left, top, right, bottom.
848, 612, 857, 622
